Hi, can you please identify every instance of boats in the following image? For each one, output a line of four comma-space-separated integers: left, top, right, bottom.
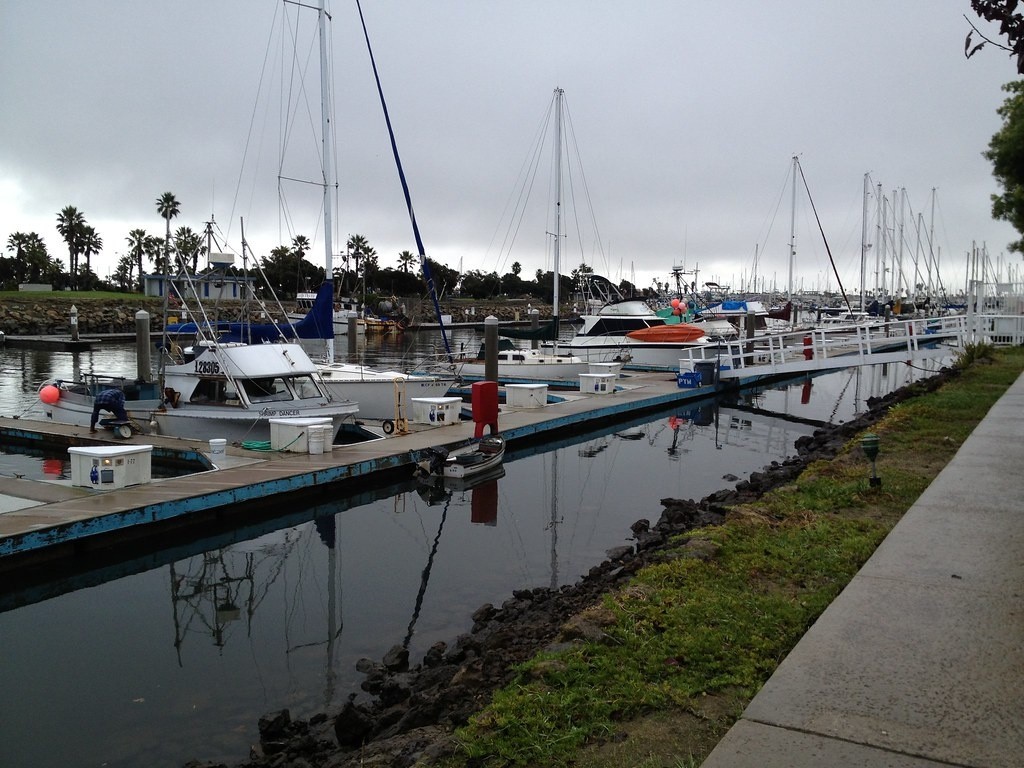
414, 461, 506, 507
541, 259, 746, 367
287, 240, 415, 335
39, 181, 359, 447
413, 434, 507, 480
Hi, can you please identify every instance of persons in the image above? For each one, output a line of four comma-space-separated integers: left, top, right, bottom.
89, 388, 131, 436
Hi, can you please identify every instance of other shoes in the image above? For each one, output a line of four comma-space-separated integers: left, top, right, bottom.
89, 429, 97, 434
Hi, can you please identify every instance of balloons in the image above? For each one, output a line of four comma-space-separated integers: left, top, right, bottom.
670, 297, 695, 316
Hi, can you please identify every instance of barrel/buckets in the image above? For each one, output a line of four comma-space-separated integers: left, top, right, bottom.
695, 362, 715, 385
307, 424, 333, 454
209, 439, 228, 459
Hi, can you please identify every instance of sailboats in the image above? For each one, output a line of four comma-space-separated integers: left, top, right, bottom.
182, 0, 474, 422
403, 85, 632, 379
570, 152, 996, 337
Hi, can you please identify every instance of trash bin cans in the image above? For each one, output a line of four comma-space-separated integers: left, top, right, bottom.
693, 360, 716, 386
694, 406, 713, 426
